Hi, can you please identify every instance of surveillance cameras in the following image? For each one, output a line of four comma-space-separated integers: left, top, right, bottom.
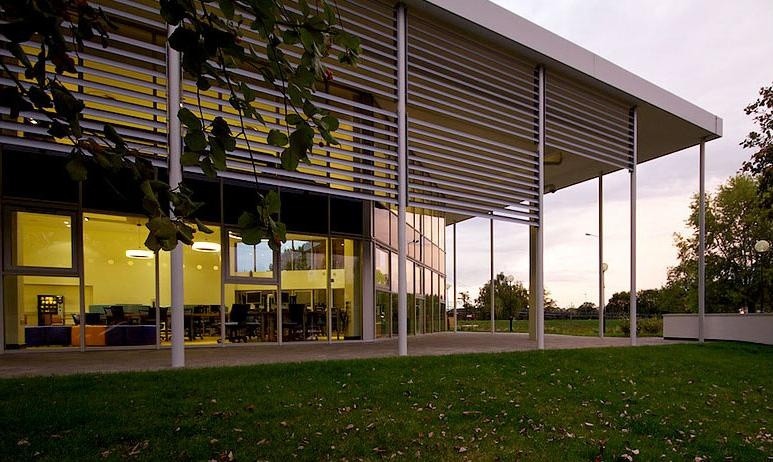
549, 184, 557, 193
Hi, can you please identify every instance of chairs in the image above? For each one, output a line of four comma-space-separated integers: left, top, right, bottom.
72, 302, 342, 343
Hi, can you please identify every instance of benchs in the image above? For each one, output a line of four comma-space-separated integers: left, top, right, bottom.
23, 324, 157, 347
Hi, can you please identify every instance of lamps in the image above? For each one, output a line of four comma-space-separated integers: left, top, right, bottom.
190, 239, 221, 253
123, 222, 155, 260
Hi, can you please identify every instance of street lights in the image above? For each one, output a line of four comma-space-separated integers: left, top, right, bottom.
601, 262, 609, 333
754, 240, 769, 313
508, 274, 515, 332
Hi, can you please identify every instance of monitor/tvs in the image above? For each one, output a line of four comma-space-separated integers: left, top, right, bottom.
86, 313, 100, 323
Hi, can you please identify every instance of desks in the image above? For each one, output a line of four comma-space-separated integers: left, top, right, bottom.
185, 311, 220, 337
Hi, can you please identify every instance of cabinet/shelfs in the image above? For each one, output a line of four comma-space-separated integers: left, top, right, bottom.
35, 293, 66, 327
